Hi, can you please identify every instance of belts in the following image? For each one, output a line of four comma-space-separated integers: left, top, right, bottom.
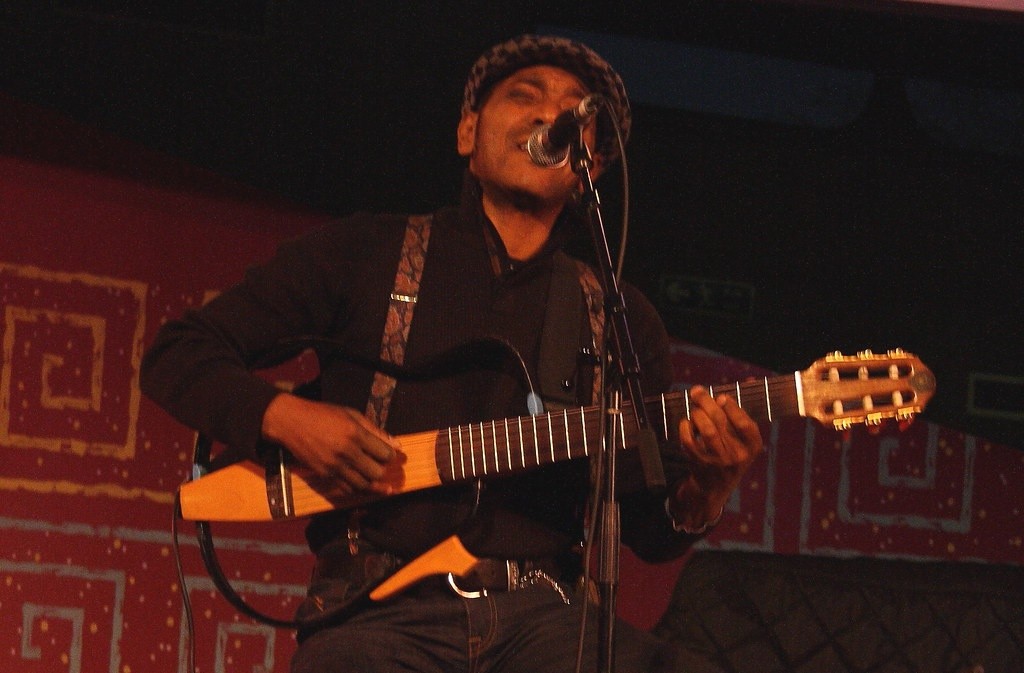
352, 546, 572, 597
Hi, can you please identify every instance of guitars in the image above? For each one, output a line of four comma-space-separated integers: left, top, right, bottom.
172, 337, 940, 629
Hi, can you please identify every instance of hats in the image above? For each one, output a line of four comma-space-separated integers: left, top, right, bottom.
463, 35, 631, 180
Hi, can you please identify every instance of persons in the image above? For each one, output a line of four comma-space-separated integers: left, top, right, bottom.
139, 33, 763, 673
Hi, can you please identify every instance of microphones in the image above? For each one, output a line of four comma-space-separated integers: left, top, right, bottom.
526, 94, 596, 170
